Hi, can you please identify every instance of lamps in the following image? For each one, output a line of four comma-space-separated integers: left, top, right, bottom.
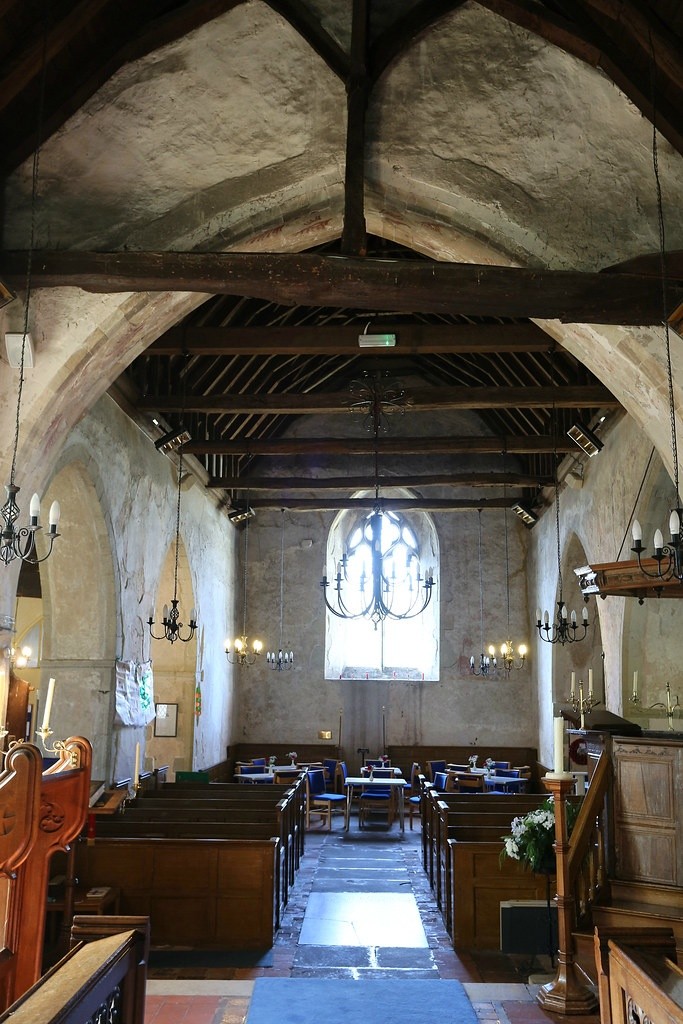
0, 85, 683, 671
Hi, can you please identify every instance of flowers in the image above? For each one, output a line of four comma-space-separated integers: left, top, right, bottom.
264, 756, 276, 763
500, 793, 594, 872
483, 758, 498, 768
468, 755, 479, 763
379, 755, 388, 763
365, 765, 376, 773
290, 752, 297, 760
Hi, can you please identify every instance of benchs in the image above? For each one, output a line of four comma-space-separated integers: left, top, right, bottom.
415, 774, 609, 962
83, 765, 312, 957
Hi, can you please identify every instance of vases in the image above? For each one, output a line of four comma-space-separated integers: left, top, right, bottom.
291, 759, 294, 767
369, 772, 374, 781
487, 768, 492, 779
268, 763, 274, 774
381, 762, 385, 768
473, 761, 477, 771
527, 835, 569, 869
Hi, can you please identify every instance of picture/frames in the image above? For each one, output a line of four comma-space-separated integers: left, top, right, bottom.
154, 702, 178, 737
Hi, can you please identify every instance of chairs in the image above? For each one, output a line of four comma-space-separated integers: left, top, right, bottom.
235, 753, 529, 831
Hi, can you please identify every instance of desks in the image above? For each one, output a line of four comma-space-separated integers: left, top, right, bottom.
345, 777, 406, 831
236, 774, 276, 784
358, 766, 403, 777
482, 772, 527, 795
275, 764, 297, 771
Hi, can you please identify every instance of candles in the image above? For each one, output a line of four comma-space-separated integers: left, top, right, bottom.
570, 668, 639, 698
42, 677, 140, 791
552, 716, 565, 773
